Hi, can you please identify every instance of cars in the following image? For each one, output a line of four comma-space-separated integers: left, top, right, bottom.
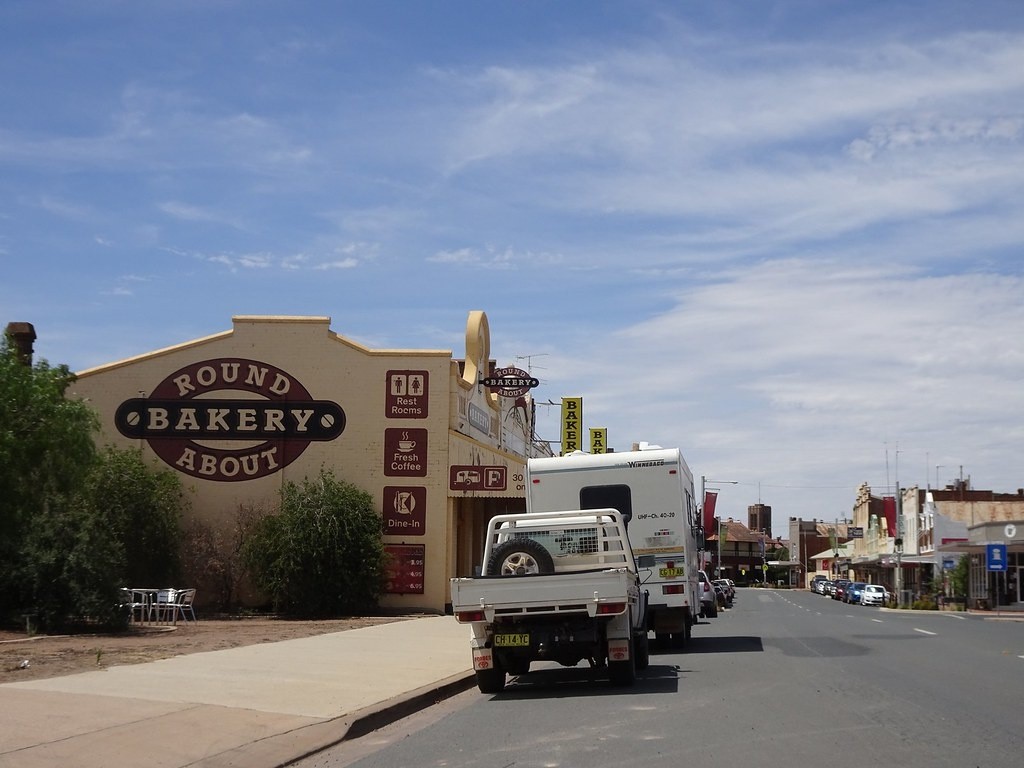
860, 585, 891, 606
810, 575, 870, 604
710, 579, 735, 607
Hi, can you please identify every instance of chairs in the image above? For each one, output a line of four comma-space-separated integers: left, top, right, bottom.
121, 589, 196, 627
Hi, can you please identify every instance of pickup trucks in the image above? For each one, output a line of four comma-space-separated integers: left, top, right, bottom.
448, 509, 649, 692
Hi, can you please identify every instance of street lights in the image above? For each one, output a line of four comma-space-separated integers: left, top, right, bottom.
700, 475, 738, 534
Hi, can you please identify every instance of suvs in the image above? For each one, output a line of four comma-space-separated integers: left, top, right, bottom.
698, 569, 718, 617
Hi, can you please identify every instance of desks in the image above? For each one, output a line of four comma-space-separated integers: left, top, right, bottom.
132, 589, 176, 625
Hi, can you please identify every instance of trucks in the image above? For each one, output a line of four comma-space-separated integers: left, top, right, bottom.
524, 442, 707, 649
